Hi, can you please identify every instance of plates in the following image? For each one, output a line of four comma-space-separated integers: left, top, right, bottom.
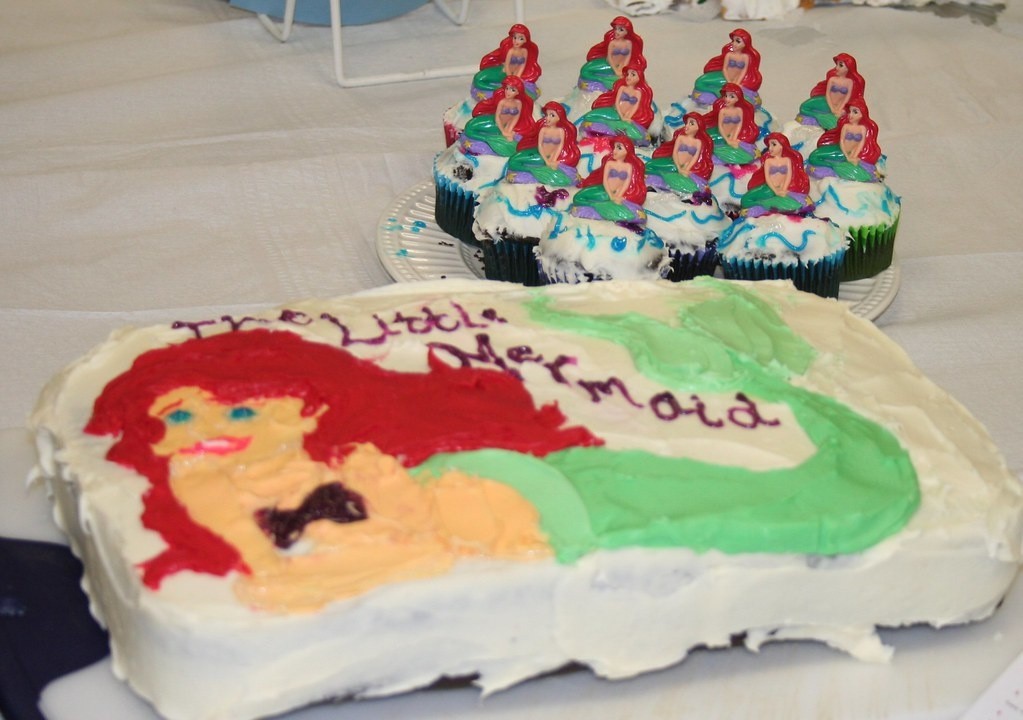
377, 178, 900, 321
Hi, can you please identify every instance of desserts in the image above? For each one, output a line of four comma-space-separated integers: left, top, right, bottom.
435, 17, 899, 301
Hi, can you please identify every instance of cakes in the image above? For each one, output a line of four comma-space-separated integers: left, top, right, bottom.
17, 276, 1021, 720
